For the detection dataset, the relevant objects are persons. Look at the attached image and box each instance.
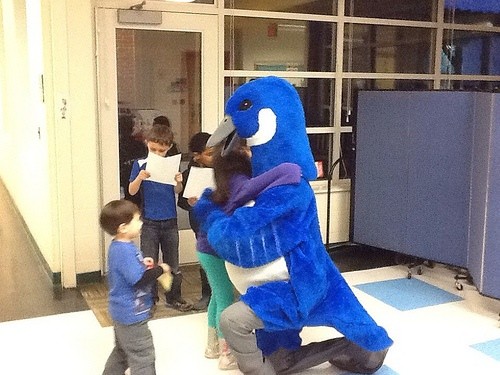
[129,124,194,312]
[119,116,149,209]
[177,132,216,309]
[100,200,171,375]
[153,116,177,156]
[196,151,301,370]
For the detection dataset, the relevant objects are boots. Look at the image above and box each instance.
[219,338,239,371]
[154,280,159,305]
[194,267,211,310]
[204,326,219,359]
[166,271,193,312]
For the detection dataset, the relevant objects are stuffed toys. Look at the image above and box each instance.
[192,76,394,375]
